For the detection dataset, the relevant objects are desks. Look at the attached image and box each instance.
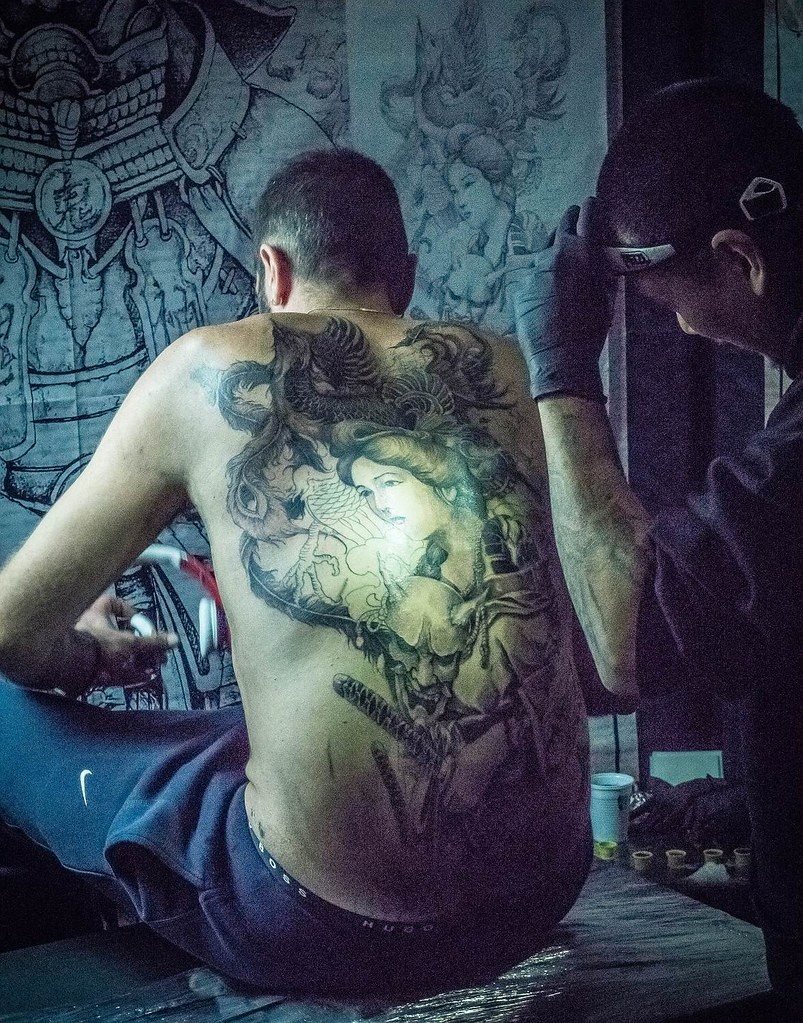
[0,858,784,1023]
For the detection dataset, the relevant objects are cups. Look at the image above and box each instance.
[631,852,653,873]
[734,847,748,872]
[589,773,633,844]
[596,840,617,861]
[703,848,723,865]
[665,849,686,868]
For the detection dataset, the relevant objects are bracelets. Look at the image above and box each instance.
[79,628,103,698]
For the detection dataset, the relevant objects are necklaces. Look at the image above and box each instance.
[307,308,390,315]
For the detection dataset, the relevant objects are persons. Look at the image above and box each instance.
[0,146,594,997]
[512,77,803,1023]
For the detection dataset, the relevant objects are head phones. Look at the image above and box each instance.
[125,544,231,662]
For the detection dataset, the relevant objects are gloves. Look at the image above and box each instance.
[506,196,621,401]
